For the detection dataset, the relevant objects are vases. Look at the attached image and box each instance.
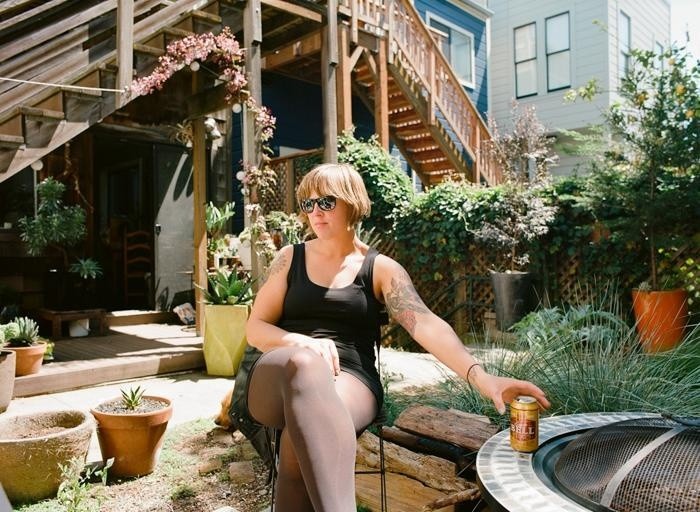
[0,350,92,507]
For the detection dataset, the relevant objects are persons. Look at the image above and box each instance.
[245,162,552,512]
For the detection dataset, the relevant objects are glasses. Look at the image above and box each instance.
[299,195,339,214]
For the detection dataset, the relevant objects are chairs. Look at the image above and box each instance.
[264,302,391,510]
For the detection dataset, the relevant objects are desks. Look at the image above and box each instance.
[477,409,699,511]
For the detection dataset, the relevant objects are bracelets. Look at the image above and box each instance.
[467,361,482,393]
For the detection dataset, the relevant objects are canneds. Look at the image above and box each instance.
[509,396,538,453]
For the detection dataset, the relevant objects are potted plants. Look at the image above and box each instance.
[194,270,259,376]
[0,310,46,373]
[468,92,562,336]
[564,30,699,353]
[91,384,174,477]
